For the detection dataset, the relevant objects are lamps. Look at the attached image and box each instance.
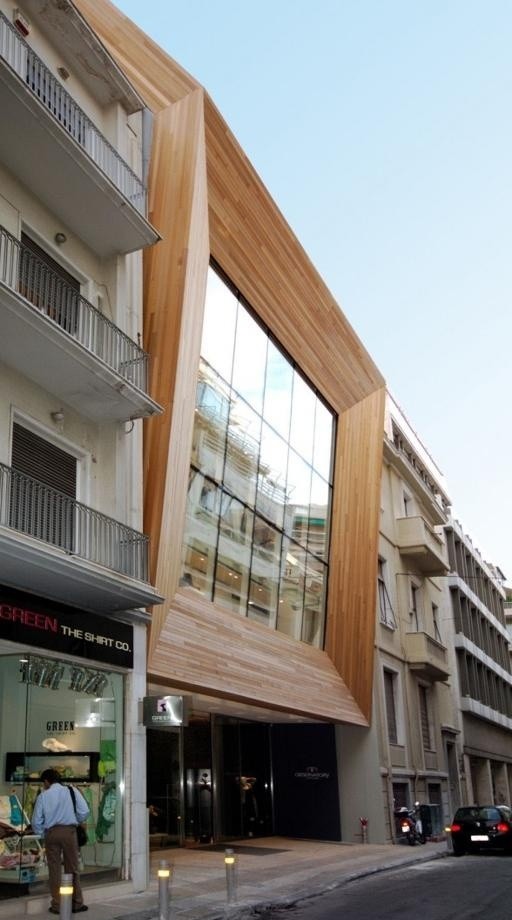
[18,653,109,700]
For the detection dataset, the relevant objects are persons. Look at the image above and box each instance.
[31,769,90,914]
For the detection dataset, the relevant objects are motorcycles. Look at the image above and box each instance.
[394,800,425,847]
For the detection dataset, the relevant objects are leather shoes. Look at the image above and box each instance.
[48,905,88,915]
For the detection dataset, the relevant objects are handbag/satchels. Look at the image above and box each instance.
[76,823,89,847]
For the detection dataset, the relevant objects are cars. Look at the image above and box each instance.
[451,803,512,855]
